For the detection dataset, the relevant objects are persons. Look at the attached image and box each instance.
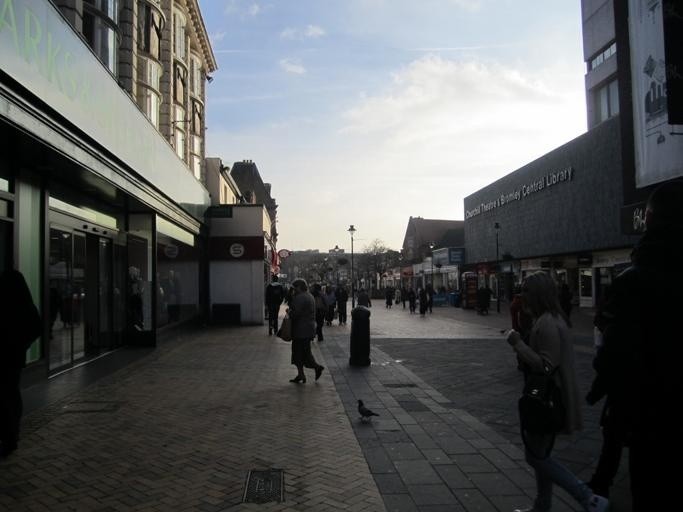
[557,282,572,324]
[383,280,495,316]
[284,279,325,384]
[579,267,631,498]
[507,285,532,373]
[508,270,612,511]
[357,287,371,308]
[284,277,347,344]
[602,178,683,511]
[262,273,282,336]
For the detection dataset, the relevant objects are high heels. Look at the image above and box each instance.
[289,374,307,384]
[315,365,324,381]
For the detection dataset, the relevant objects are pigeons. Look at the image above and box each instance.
[356,400,380,424]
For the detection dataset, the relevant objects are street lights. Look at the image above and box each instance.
[347,225,357,310]
[397,253,402,288]
[313,263,317,281]
[306,265,310,286]
[61,232,68,327]
[334,245,340,287]
[494,222,501,313]
[429,242,434,290]
[323,256,327,285]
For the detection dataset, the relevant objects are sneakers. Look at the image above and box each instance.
[586,495,609,511]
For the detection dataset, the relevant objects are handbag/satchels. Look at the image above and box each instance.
[518,357,565,433]
[276,308,292,342]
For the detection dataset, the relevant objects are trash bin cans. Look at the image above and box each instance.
[349,307,370,366]
[449,293,459,307]
